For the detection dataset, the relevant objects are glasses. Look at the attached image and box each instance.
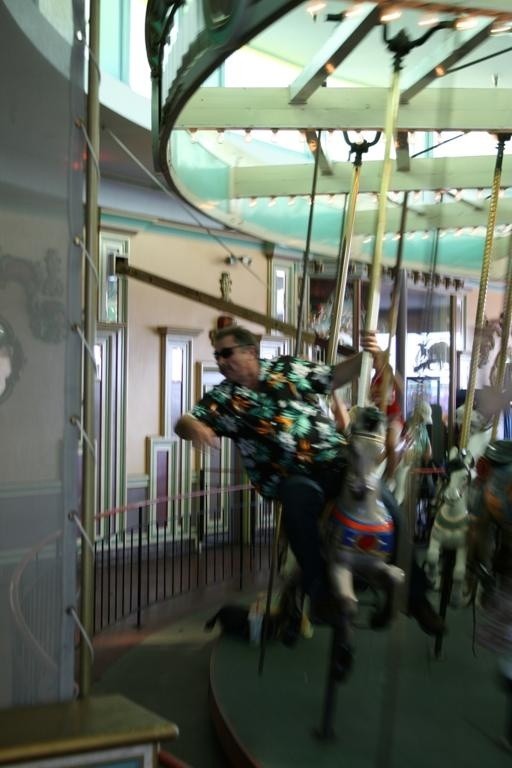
[214,343,253,361]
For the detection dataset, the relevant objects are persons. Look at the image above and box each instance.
[174,325,450,636]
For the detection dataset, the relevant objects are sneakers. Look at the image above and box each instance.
[403,595,448,636]
[330,627,355,683]
